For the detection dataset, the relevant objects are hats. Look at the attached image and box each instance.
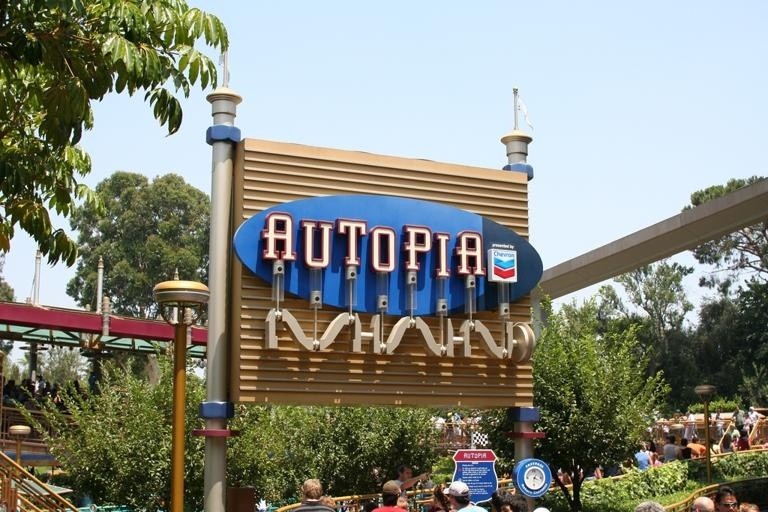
[383,480,400,495]
[444,482,470,496]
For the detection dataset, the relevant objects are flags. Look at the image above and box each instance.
[516,97,536,132]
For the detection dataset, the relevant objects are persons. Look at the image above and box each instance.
[320,479,560,512]
[558,392,768,511]
[430,408,501,446]
[393,463,432,493]
[0,369,102,414]
[289,477,337,512]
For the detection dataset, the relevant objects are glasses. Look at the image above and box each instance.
[720,502,737,507]
